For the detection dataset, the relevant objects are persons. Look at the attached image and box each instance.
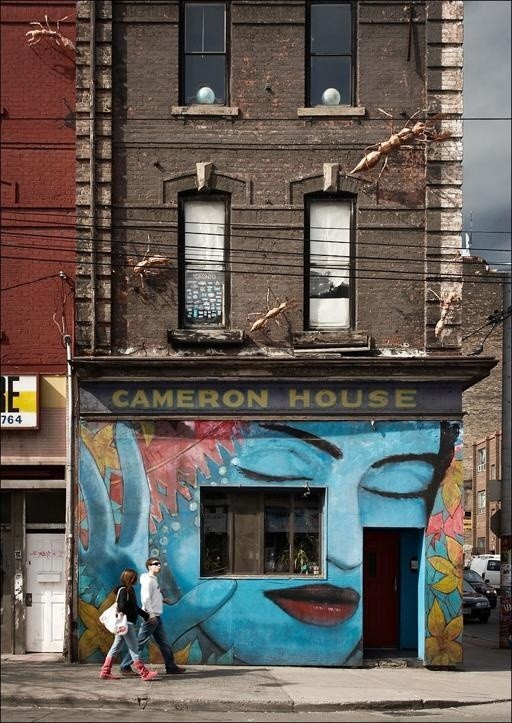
[98,569,158,681]
[119,557,186,676]
[78,420,457,668]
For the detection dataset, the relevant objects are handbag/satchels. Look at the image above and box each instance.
[99,603,128,635]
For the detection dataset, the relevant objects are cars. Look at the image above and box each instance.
[265,545,292,571]
[461,553,502,622]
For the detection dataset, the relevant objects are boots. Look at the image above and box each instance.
[100,657,120,680]
[133,660,158,681]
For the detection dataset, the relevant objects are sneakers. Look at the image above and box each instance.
[121,671,137,676]
[166,668,186,674]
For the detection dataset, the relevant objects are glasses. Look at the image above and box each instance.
[150,563,160,566]
